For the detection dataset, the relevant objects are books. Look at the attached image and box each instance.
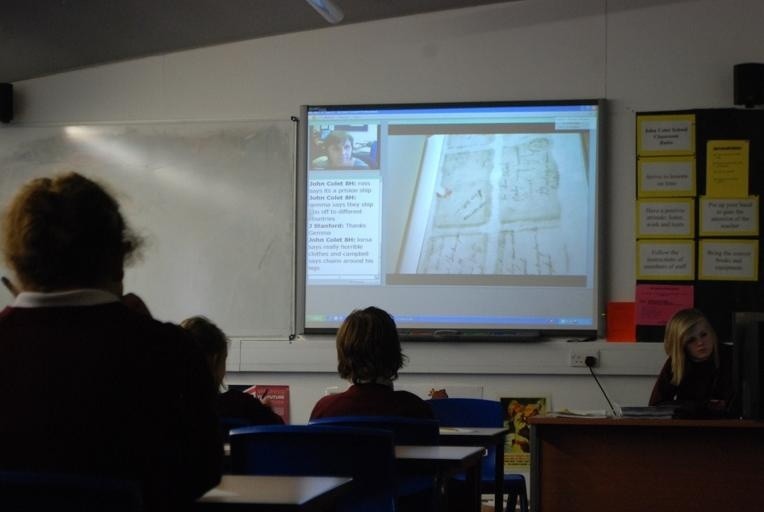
[616,404,672,420]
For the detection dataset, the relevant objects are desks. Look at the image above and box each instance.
[395,445,485,511]
[198,475,354,512]
[438,427,513,512]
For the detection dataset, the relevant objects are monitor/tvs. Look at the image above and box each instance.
[729,308,764,419]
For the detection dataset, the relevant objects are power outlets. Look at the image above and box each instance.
[568,350,601,368]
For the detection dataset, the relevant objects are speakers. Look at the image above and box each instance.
[733,62,764,108]
[0,82,14,124]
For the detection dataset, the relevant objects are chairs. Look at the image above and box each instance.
[306,415,441,512]
[426,399,528,510]
[229,424,395,512]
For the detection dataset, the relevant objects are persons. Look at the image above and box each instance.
[0,168,223,511]
[647,307,736,412]
[178,312,284,439]
[311,306,480,511]
[313,129,370,168]
